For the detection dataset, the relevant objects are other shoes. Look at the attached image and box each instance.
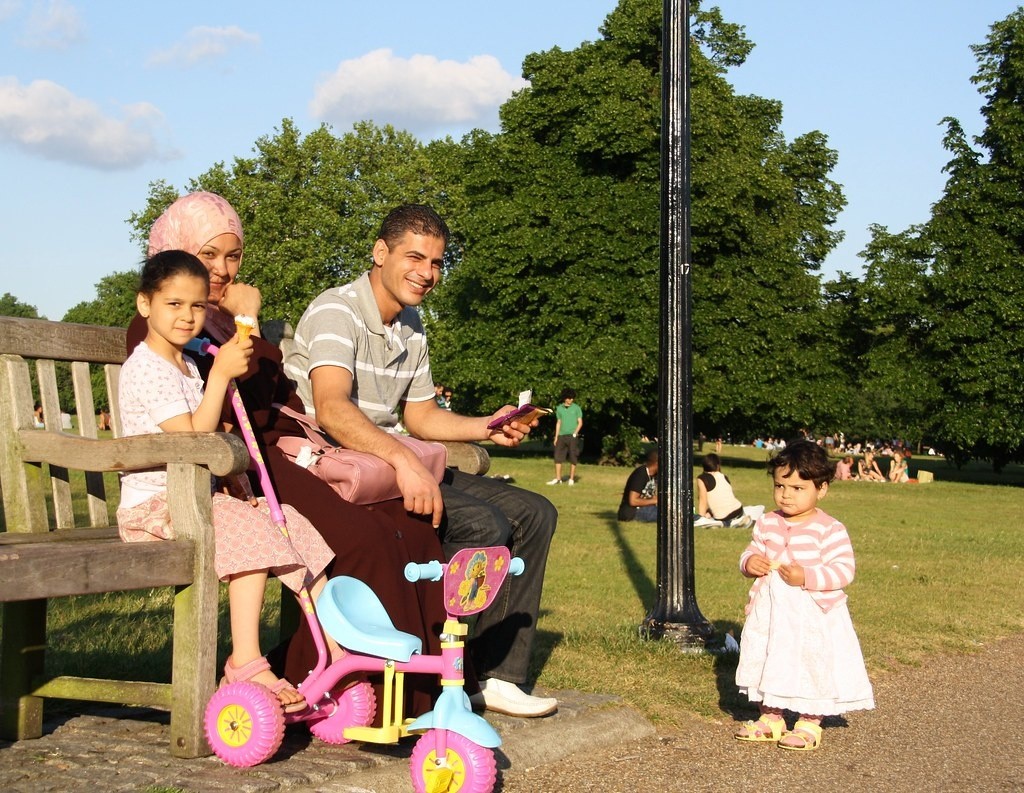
[468,677,557,717]
[546,479,563,485]
[568,479,574,486]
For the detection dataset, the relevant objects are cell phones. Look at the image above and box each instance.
[487,403,550,432]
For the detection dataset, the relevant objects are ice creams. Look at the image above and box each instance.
[235,314,255,342]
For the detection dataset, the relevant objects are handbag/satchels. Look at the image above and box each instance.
[275,432,447,505]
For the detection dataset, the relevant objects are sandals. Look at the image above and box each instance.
[734,715,787,741]
[219,655,307,712]
[777,720,822,751]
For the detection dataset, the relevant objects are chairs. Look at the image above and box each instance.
[0,312,494,767]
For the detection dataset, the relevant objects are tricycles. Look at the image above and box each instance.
[180,336,524,793]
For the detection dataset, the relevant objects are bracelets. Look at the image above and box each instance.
[573,434,577,438]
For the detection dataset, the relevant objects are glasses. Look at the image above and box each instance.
[445,395,451,398]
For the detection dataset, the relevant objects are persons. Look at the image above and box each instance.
[116,250,366,712]
[736,440,876,751]
[125,192,483,728]
[641,432,658,443]
[751,427,945,483]
[715,431,722,453]
[284,202,558,717]
[698,432,706,452]
[546,387,582,486]
[34,403,110,431]
[434,381,453,412]
[617,447,746,530]
